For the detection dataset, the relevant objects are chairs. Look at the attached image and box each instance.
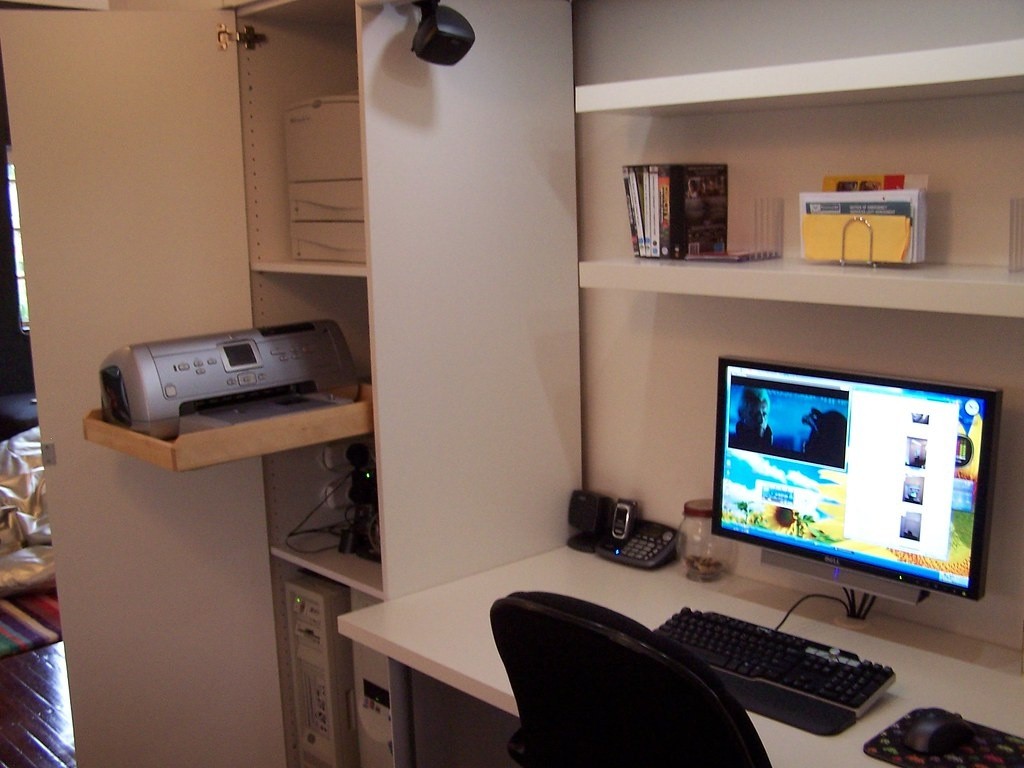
[489,590,772,768]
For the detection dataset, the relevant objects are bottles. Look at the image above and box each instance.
[676,499,731,581]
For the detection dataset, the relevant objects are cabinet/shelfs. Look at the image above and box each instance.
[0,0,585,767]
[574,40,1024,318]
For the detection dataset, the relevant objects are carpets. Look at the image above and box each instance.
[0,586,62,660]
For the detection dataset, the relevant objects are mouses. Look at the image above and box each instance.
[904,709,975,755]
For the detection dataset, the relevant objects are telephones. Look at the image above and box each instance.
[595,498,686,571]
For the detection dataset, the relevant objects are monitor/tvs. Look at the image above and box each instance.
[712,355,1003,603]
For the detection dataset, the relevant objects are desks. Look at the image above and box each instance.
[336,546,1024,768]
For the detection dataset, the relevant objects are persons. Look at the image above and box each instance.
[728,387,772,447]
[801,408,847,463]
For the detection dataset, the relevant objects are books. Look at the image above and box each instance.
[622,164,728,258]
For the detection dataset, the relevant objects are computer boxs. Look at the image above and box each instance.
[286,580,395,768]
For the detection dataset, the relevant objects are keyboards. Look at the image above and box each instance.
[653,607,896,739]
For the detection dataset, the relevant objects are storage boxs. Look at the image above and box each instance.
[286,96,366,265]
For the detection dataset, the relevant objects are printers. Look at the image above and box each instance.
[97,318,360,443]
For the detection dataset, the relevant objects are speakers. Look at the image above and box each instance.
[567,491,614,554]
